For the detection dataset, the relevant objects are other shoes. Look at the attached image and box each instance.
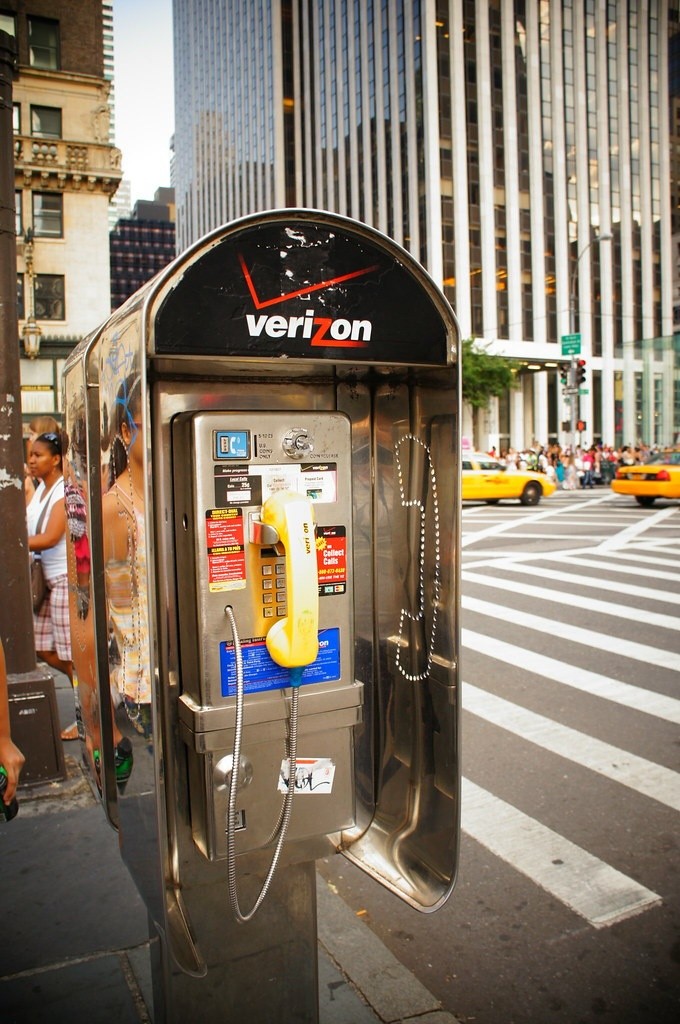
[94,738,132,784]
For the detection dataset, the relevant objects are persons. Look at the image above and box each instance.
[0,638,26,806]
[63,370,151,800]
[490,441,680,489]
[22,416,65,508]
[22,433,78,740]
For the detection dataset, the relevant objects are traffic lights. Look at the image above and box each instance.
[562,421,570,431]
[576,420,586,431]
[560,365,567,384]
[576,360,586,383]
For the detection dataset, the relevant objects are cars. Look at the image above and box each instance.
[462,453,556,506]
[611,448,680,506]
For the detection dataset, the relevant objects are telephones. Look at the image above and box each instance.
[169,406,358,710]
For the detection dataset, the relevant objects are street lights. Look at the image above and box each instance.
[568,233,614,490]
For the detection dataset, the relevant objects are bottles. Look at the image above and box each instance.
[0,766,19,823]
[93,738,133,795]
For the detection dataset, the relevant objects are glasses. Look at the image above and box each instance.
[41,432,61,447]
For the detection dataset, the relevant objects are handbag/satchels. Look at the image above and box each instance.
[30,559,45,615]
